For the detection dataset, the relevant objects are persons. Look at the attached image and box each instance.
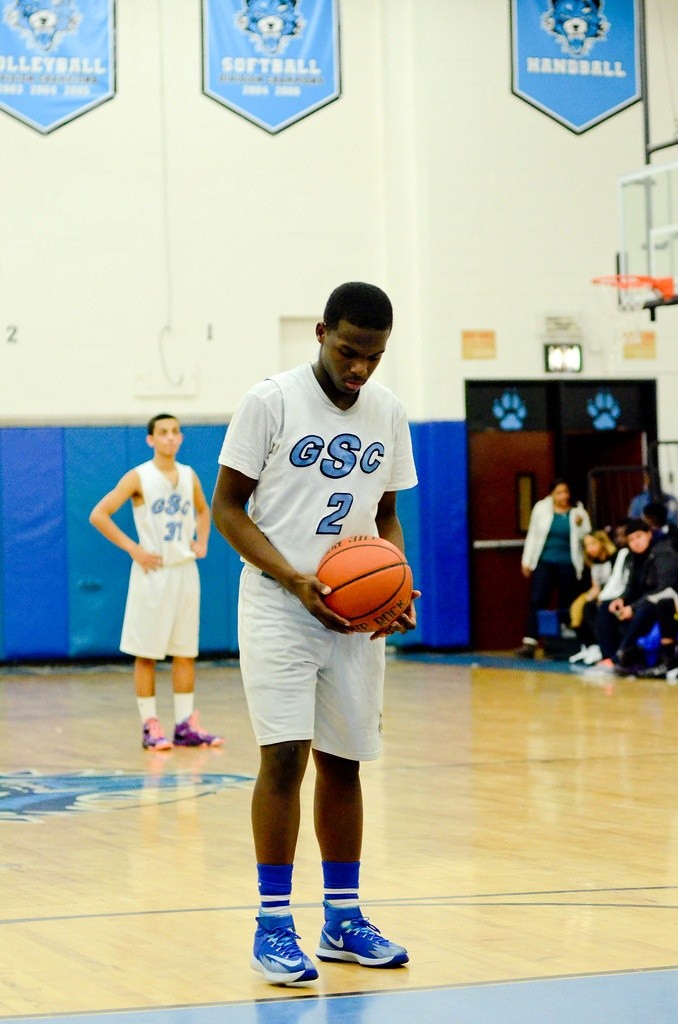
[520,476,678,684]
[88,413,223,753]
[209,281,422,985]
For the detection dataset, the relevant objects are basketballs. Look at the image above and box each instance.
[318,534,414,633]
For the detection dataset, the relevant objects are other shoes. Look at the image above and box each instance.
[522,646,636,681]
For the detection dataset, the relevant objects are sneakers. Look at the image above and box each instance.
[172,710,222,747]
[249,909,318,986]
[315,900,409,967]
[143,718,173,751]
